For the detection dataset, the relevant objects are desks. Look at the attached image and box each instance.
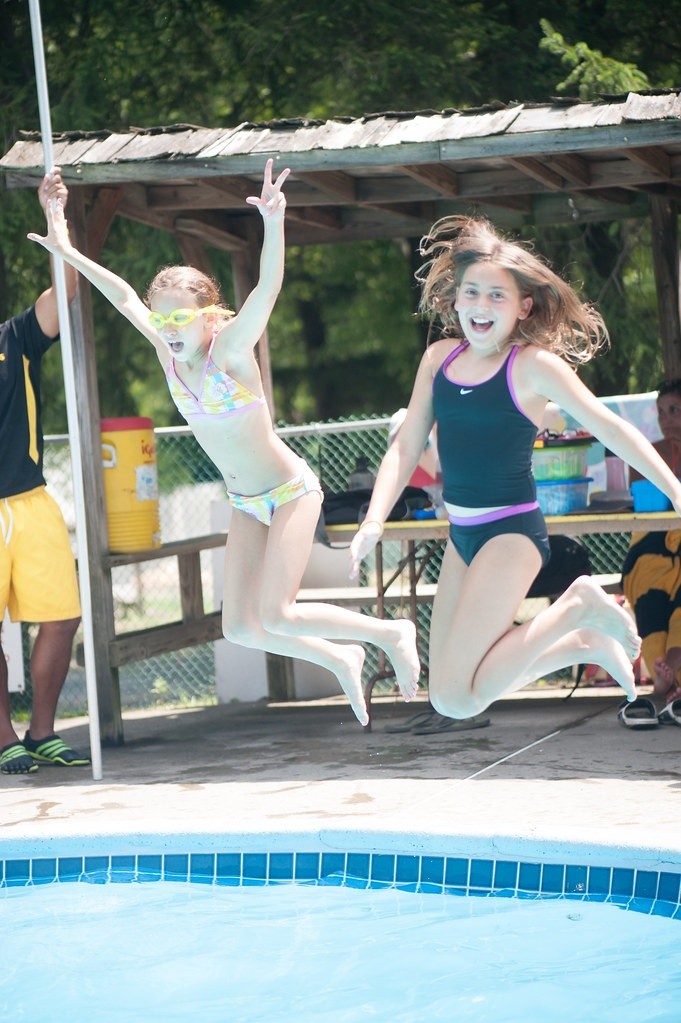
[309,510,681,733]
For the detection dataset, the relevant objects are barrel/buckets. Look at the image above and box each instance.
[100,417,162,553]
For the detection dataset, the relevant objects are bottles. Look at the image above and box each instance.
[604,448,627,493]
[351,459,372,489]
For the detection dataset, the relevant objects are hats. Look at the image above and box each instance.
[386,408,408,447]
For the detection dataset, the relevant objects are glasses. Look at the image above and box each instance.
[149,305,236,330]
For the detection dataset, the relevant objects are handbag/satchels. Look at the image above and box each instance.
[314,484,437,549]
[526,534,592,598]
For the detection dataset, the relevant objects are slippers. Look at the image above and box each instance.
[411,712,490,735]
[384,711,436,734]
[657,698,681,727]
[616,697,660,727]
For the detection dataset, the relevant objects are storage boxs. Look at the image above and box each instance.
[530,443,596,515]
[627,480,671,514]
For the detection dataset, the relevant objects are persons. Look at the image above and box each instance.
[620,379,680,702]
[0,166,92,774]
[28,159,421,727]
[350,216,680,719]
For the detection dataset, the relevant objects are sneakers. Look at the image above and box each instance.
[0,741,39,774]
[21,729,91,767]
